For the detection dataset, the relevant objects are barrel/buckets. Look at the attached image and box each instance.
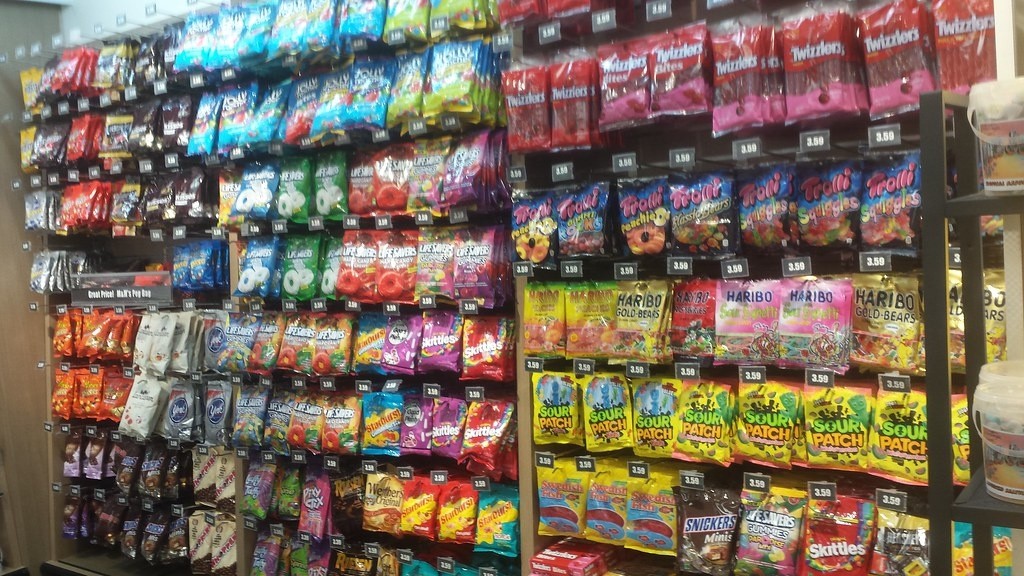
[966,77,1024,195]
[972,360,1024,505]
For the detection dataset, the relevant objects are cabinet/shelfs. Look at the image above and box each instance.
[917,91,1024,576]
